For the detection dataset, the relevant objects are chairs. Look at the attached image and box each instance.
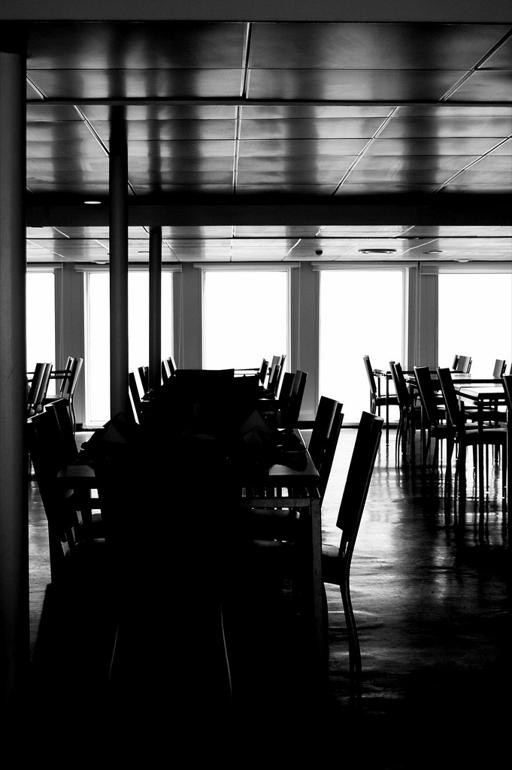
[25,355,381,705]
[361,356,509,537]
